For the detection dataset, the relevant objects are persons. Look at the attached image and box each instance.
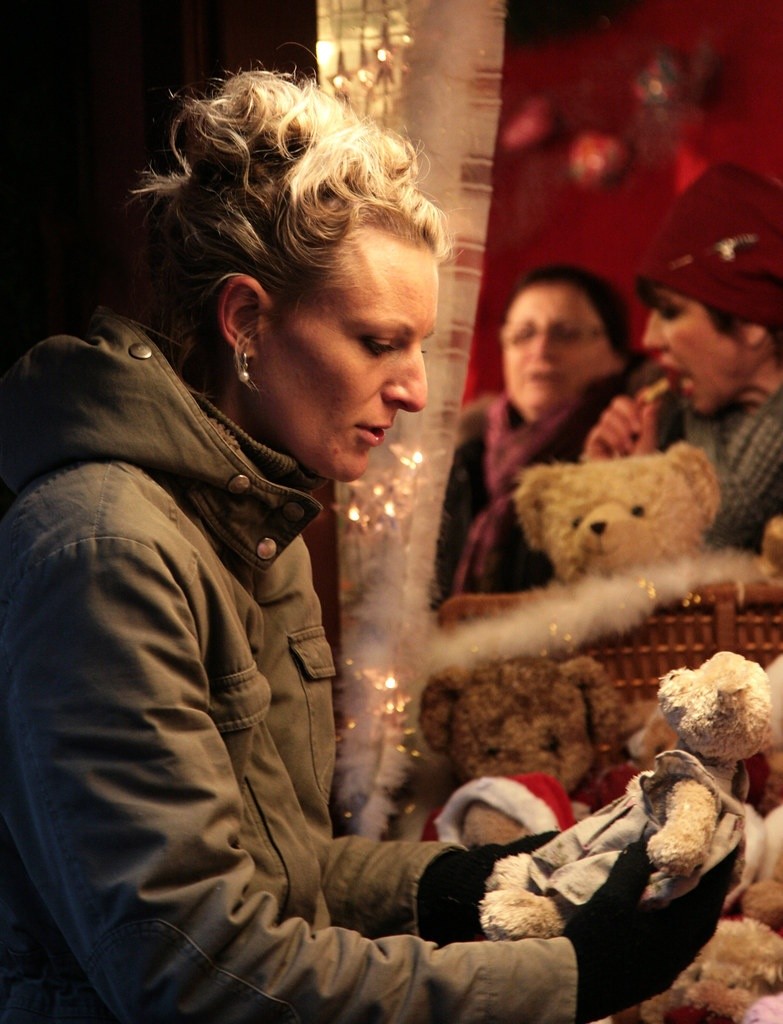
[575,159,783,561]
[421,258,648,614]
[0,70,741,1024]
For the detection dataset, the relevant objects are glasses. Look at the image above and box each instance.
[500,320,605,348]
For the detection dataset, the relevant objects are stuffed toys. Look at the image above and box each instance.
[418,653,783,1024]
[511,440,725,601]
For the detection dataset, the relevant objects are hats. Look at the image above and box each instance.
[633,162,783,329]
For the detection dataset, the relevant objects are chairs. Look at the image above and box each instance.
[445,576,783,704]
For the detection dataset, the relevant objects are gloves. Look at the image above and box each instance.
[557,839,740,1024]
[413,830,567,943]
